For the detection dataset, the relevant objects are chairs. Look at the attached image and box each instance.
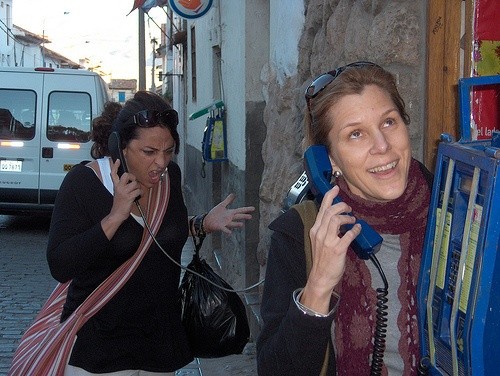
[20,110,34,130]
[56,110,79,128]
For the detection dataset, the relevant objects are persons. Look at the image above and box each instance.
[256,62,445,376]
[47,89,256,376]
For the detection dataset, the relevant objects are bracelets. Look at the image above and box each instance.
[194,213,209,236]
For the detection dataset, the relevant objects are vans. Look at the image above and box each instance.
[0,66,115,216]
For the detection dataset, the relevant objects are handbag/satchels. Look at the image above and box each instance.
[10,280,75,376]
[179,217,249,358]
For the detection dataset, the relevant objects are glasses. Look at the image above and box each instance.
[304,61,382,127]
[120,110,180,131]
